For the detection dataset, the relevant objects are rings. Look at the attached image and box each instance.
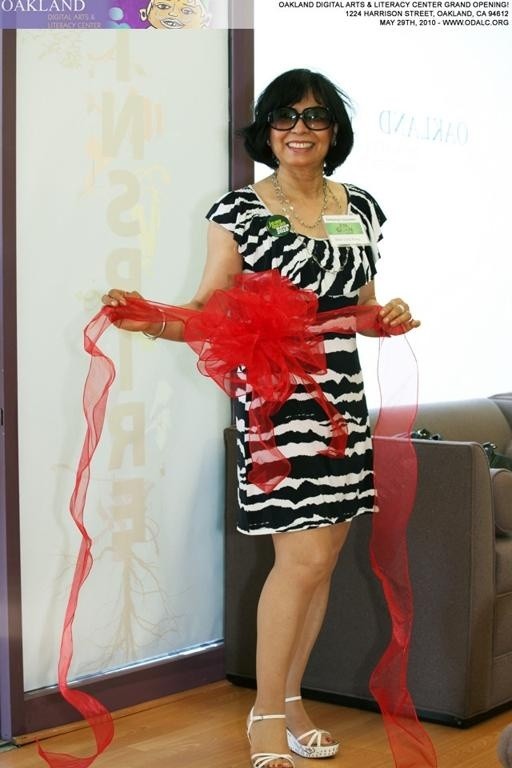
[398,303,405,313]
[406,311,411,320]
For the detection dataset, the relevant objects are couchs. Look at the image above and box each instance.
[222,394,511,730]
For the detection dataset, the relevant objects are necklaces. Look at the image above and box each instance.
[271,172,350,274]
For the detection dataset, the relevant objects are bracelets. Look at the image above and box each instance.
[142,308,168,339]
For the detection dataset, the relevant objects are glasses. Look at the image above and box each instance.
[264,105,335,131]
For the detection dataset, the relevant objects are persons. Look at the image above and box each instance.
[100,69,421,768]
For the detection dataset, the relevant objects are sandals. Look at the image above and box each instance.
[247,706,296,768]
[286,696,340,760]
